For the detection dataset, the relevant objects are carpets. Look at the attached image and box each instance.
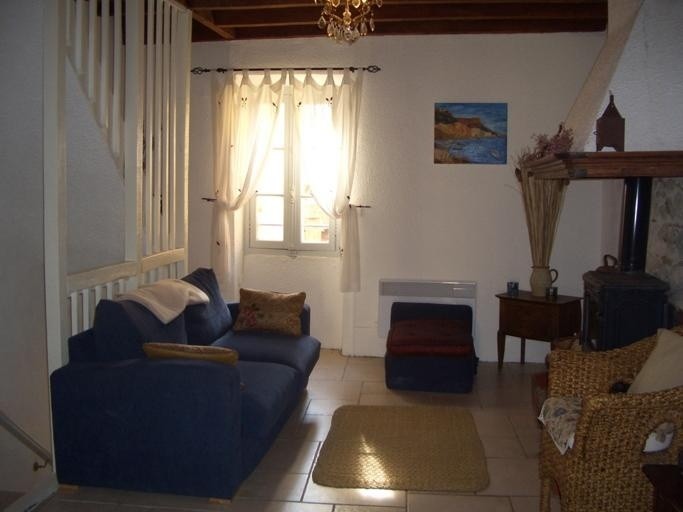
[313,403,490,493]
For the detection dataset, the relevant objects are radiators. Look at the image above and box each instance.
[378,278,478,338]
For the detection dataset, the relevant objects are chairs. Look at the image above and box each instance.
[539,327,683,512]
[384,302,480,393]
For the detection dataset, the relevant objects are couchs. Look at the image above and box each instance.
[50,268,322,500]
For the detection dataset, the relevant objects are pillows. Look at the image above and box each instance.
[141,342,245,392]
[627,328,683,395]
[233,287,309,338]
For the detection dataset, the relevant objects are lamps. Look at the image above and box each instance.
[313,0,387,46]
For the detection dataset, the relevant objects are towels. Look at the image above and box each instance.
[112,278,211,324]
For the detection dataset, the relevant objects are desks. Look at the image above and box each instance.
[642,465,683,512]
[493,288,585,369]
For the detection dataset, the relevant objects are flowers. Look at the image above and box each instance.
[515,129,574,264]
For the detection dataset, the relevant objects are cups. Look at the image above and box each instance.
[506,281,518,297]
[545,286,557,301]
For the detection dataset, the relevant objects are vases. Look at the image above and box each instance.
[530,266,558,298]
